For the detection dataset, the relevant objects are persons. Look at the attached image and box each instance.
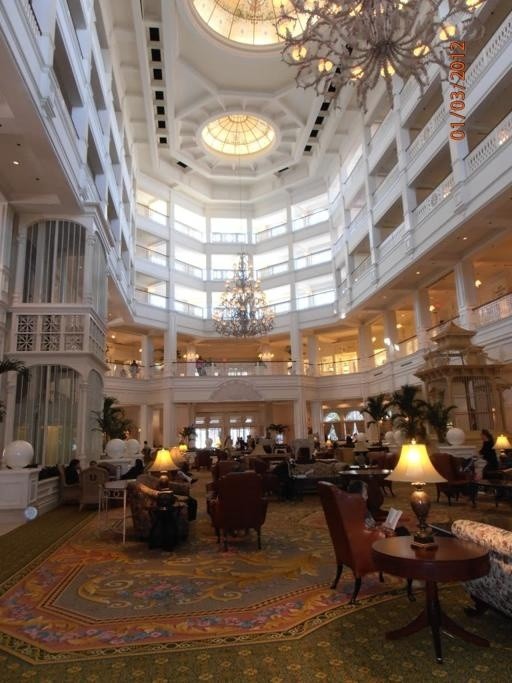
[129,359,139,377]
[120,459,145,479]
[476,428,498,493]
[222,434,256,460]
[90,460,98,466]
[196,355,207,375]
[347,480,411,537]
[143,440,150,448]
[64,458,83,483]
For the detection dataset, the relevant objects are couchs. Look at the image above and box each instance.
[451,520,512,620]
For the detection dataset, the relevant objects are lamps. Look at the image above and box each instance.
[491,434,512,470]
[383,437,449,551]
[210,121,277,339]
[147,448,182,496]
[271,0,487,115]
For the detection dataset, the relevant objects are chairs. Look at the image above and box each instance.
[130,462,190,542]
[429,453,476,506]
[57,461,121,513]
[207,473,267,552]
[206,461,244,528]
[366,452,396,469]
[317,481,416,603]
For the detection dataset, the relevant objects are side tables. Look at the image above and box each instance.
[372,536,490,664]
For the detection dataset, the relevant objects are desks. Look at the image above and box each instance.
[334,469,395,497]
[97,478,137,545]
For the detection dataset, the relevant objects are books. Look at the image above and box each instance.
[381,506,403,530]
